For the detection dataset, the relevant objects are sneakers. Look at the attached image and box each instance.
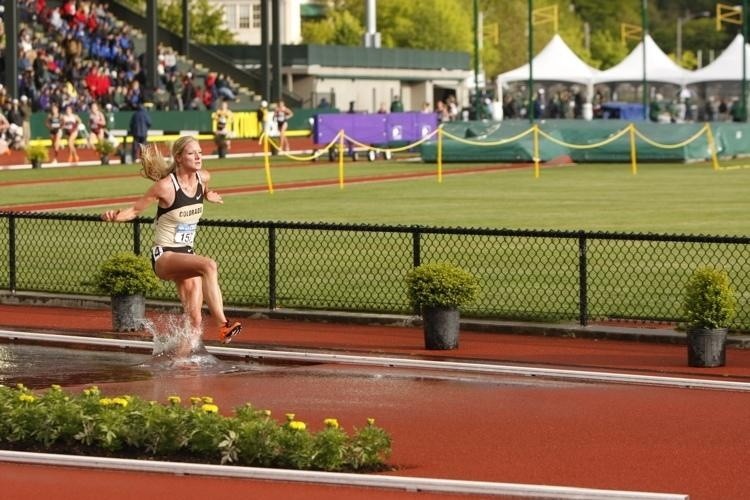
[218,320,244,345]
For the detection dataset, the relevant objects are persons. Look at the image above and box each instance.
[0,0,273,163]
[348,102,357,156]
[390,96,402,113]
[378,104,386,114]
[422,85,604,122]
[100,135,242,359]
[318,99,329,108]
[649,93,749,124]
[271,99,294,152]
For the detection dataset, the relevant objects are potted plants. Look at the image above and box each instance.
[95,138,114,165]
[24,142,48,169]
[95,251,160,331]
[675,267,737,369]
[404,261,480,349]
